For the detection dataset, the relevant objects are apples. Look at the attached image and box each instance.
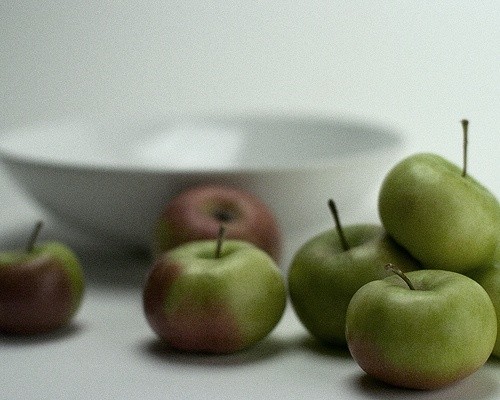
[151,184,281,262]
[286,119,499,390]
[0,219,85,337]
[142,224,287,356]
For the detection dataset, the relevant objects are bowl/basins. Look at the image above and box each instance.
[0,105,404,254]
